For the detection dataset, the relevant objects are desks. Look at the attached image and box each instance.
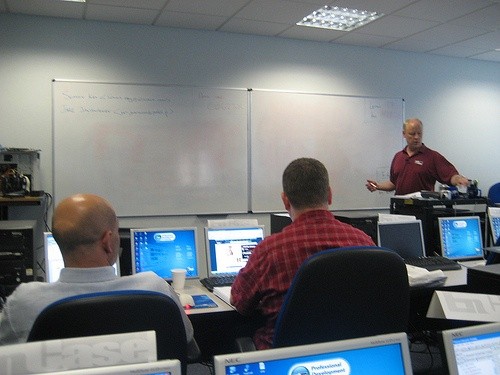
[172,279,248,375]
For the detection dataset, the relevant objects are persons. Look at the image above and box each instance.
[231,157,379,350]
[366,118,469,214]
[0,194,193,347]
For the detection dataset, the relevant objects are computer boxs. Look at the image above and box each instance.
[0,220,37,311]
[390,194,487,257]
[271,213,292,236]
[333,212,378,248]
[467,263,500,297]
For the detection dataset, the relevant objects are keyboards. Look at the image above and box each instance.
[200,276,239,291]
[403,255,459,271]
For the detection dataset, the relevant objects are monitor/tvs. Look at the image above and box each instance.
[441,322,500,375]
[38,359,182,375]
[438,216,483,260]
[489,215,500,245]
[376,219,426,257]
[44,232,120,284]
[130,227,199,281]
[204,225,264,277]
[213,333,414,375]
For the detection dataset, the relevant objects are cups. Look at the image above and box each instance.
[171,269,186,292]
[467,186,481,198]
[445,187,458,200]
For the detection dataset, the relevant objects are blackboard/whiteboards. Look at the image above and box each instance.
[250,88,406,213]
[52,80,250,217]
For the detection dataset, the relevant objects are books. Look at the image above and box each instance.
[213,287,239,312]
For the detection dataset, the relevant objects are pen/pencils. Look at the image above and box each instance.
[370,182,380,190]
